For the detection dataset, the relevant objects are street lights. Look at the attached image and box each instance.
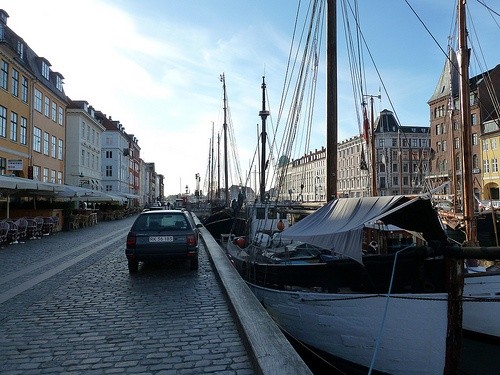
[185,185,189,209]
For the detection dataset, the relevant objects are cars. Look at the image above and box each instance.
[140,199,175,212]
[125,209,203,275]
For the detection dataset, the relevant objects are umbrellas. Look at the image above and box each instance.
[0,174,136,247]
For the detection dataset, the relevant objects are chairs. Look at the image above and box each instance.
[0,205,143,248]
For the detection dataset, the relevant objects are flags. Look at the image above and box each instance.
[362,104,370,144]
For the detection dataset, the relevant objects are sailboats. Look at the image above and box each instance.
[420,1,500,362]
[236,0,469,373]
[199,0,322,244]
